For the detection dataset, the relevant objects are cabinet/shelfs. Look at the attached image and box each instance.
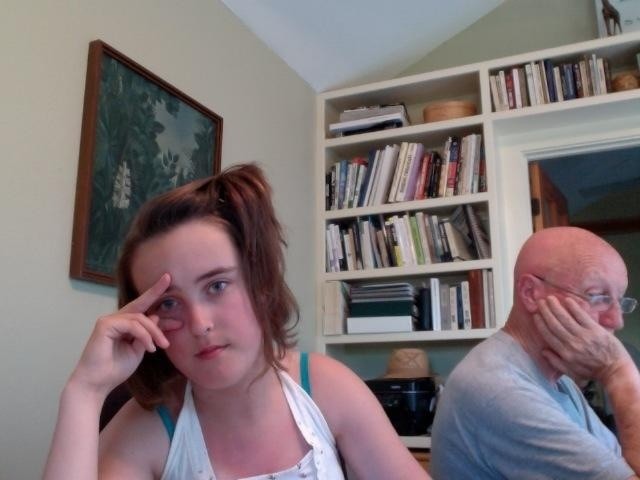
[316,30,640,451]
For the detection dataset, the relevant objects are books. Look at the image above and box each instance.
[490,53,613,111]
[322,101,495,335]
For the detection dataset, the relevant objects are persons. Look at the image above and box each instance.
[430,224,640,480]
[43,158,433,480]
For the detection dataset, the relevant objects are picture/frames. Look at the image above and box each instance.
[70,39,224,285]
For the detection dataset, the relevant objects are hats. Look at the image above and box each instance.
[385,348,438,380]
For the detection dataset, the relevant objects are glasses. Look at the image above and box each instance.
[531,274,637,314]
